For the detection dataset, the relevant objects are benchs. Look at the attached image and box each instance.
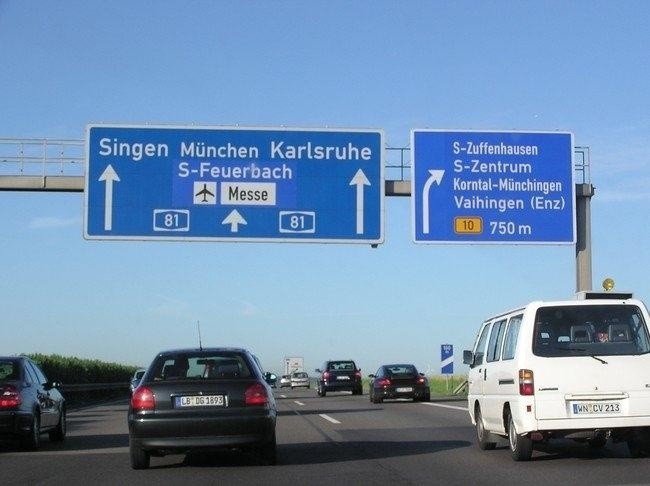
[546,324,636,357]
[163,364,239,380]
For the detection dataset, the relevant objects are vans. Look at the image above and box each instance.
[462,293,650,461]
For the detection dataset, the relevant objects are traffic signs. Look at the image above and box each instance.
[410,128,577,246]
[82,123,385,243]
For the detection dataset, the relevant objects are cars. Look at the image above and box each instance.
[128,348,277,469]
[280,356,430,403]
[1,356,67,450]
